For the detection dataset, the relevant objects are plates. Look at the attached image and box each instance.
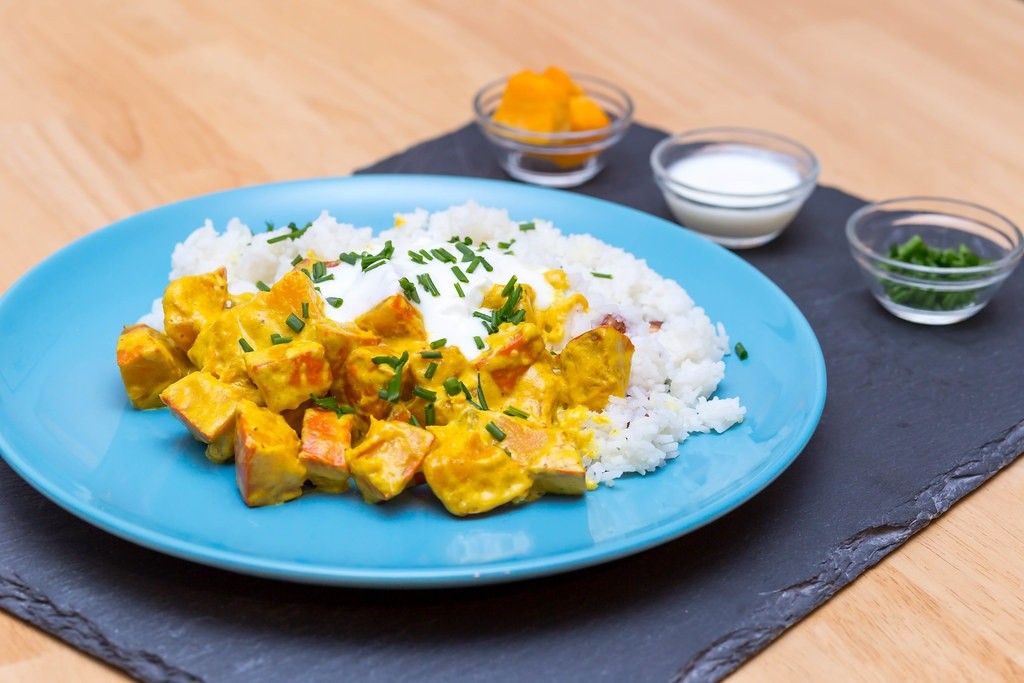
[0,175,829,586]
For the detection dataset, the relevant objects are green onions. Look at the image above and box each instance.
[241,221,749,442]
[876,234,999,310]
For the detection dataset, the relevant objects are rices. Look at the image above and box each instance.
[137,201,746,485]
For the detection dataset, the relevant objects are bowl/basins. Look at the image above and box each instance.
[473,68,635,188]
[844,196,1024,325]
[650,126,820,249]
